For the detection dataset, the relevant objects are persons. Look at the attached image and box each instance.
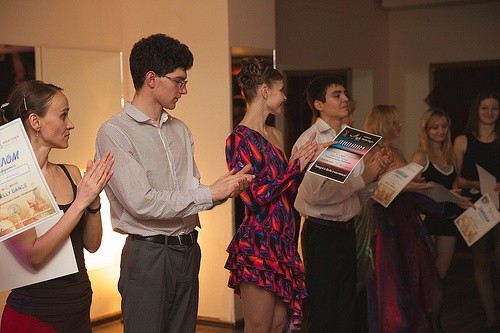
[452,90,500,333]
[225,54,338,333]
[409,107,480,289]
[361,105,445,333]
[93,33,257,333]
[290,75,393,333]
[0,79,116,332]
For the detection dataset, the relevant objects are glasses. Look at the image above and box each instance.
[145,70,187,88]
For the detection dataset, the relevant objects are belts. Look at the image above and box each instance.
[305,215,354,232]
[127,230,198,245]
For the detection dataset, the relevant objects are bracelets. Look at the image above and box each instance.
[86,204,101,213]
